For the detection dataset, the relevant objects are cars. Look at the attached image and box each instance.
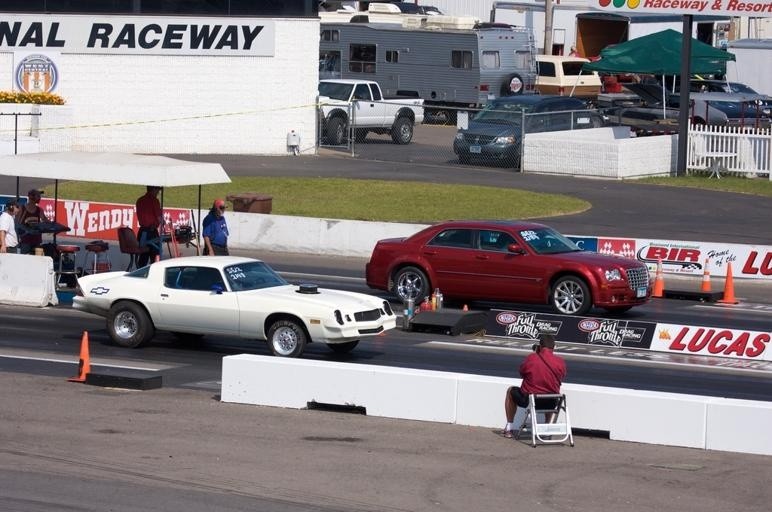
[361,219,656,325]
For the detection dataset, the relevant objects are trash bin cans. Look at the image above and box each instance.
[226,193,273,214]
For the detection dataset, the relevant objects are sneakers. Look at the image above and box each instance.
[498,429,514,438]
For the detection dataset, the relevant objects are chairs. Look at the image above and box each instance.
[117,227,149,272]
[516,394,575,448]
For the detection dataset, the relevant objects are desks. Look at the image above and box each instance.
[695,152,738,179]
[15,222,70,244]
[159,232,199,258]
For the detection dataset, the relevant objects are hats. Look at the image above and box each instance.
[147,185,164,191]
[27,189,45,197]
[5,197,21,208]
[209,198,225,209]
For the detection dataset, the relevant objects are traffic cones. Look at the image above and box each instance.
[68,329,97,381]
[650,257,666,297]
[713,260,745,306]
[701,258,712,294]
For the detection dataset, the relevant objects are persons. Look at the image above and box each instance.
[568,46,582,57]
[0,202,21,254]
[202,199,230,256]
[500,334,567,438]
[14,189,50,255]
[136,186,163,268]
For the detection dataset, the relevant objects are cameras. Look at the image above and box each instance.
[532,344,540,351]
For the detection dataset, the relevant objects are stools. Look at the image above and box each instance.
[81,240,111,277]
[55,245,81,292]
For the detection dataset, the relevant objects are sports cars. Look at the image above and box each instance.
[72,255,402,357]
